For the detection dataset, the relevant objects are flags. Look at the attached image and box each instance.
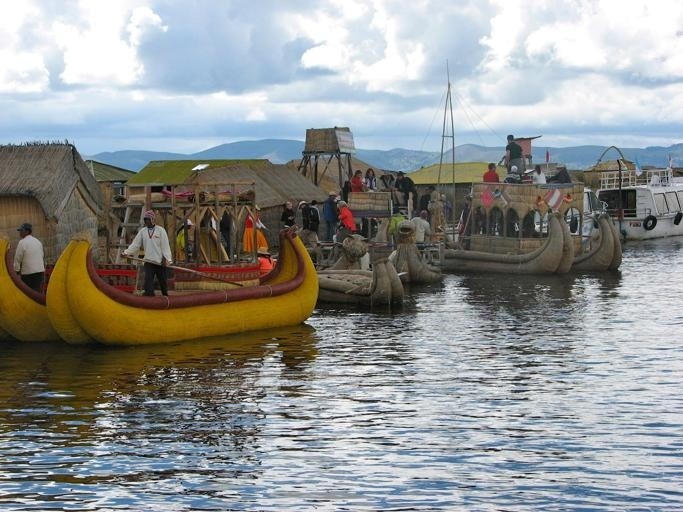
[635,158,643,177]
[667,154,672,171]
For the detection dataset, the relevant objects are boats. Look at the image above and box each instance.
[43,228,319,348]
[305,258,405,309]
[596,187,682,242]
[0,235,61,344]
[468,214,623,272]
[312,233,367,270]
[391,222,443,281]
[440,214,573,275]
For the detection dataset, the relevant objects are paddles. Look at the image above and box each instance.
[345,272,409,293]
[125,255,245,288]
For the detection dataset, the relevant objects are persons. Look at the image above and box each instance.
[456,134,571,240]
[120,209,172,296]
[279,166,453,259]
[175,204,269,264]
[13,223,44,292]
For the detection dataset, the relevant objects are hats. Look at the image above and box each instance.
[382,170,391,175]
[395,171,406,174]
[257,247,270,254]
[556,163,564,168]
[17,223,32,231]
[298,201,307,209]
[329,192,337,196]
[144,211,155,225]
[183,219,193,225]
[511,166,517,172]
